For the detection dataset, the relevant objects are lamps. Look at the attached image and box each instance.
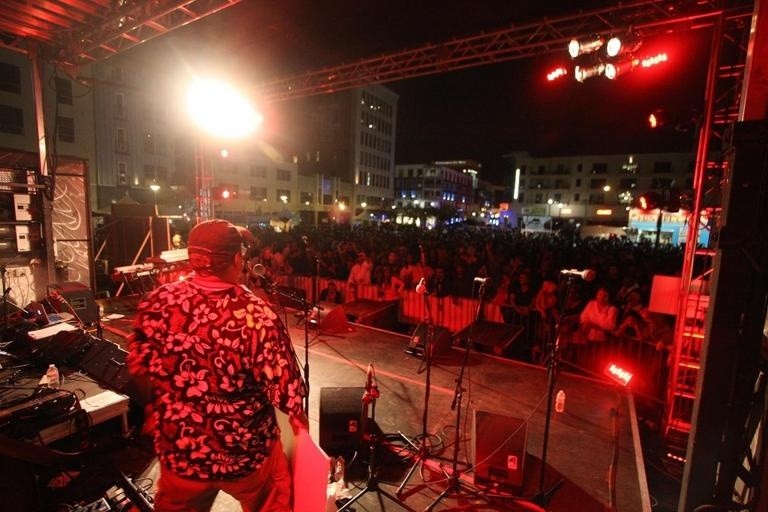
[567,30,644,83]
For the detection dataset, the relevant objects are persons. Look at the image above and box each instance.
[258,219,707,359]
[127,217,310,512]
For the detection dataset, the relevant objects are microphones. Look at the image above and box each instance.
[253,264,272,284]
[415,277,426,294]
[473,276,492,286]
[561,268,596,281]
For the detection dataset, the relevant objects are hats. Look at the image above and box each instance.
[187,219,254,258]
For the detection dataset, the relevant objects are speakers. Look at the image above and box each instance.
[56,281,99,326]
[319,386,371,456]
[472,409,529,496]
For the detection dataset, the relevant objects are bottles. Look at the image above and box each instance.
[46,364,60,389]
[555,389,567,413]
[26,169,36,195]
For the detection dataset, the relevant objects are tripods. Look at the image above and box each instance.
[533,357,564,507]
[423,384,511,512]
[304,262,346,347]
[334,397,419,512]
[396,318,472,496]
